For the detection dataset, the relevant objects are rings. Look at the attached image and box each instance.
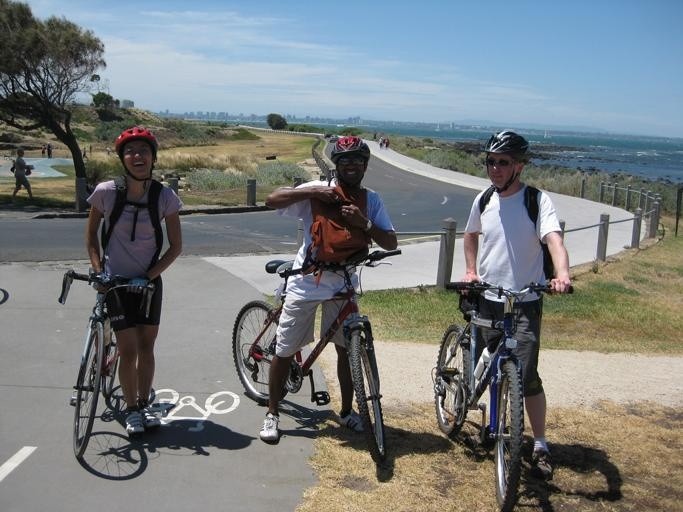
[334,196,338,200]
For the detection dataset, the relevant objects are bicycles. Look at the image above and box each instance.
[232,249,399,464]
[431,280,573,511]
[57,259,156,459]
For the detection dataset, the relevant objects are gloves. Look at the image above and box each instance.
[127,275,150,294]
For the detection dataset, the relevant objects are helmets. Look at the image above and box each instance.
[116,127,159,154]
[331,136,370,162]
[485,131,531,162]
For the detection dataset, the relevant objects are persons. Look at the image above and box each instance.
[82,148,88,161]
[12,149,34,198]
[260,136,397,444]
[462,131,571,479]
[47,142,53,158]
[84,128,184,436]
[374,134,390,150]
[41,144,46,158]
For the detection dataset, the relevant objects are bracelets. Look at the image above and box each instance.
[465,270,478,275]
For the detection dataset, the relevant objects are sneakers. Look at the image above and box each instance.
[141,407,161,428]
[260,412,279,442]
[125,411,145,434]
[339,408,365,431]
[532,449,553,480]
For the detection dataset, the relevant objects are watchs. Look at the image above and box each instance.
[363,219,372,232]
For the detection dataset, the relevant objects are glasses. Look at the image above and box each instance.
[486,157,511,166]
[338,155,364,166]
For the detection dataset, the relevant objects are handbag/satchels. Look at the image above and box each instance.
[312,204,368,262]
